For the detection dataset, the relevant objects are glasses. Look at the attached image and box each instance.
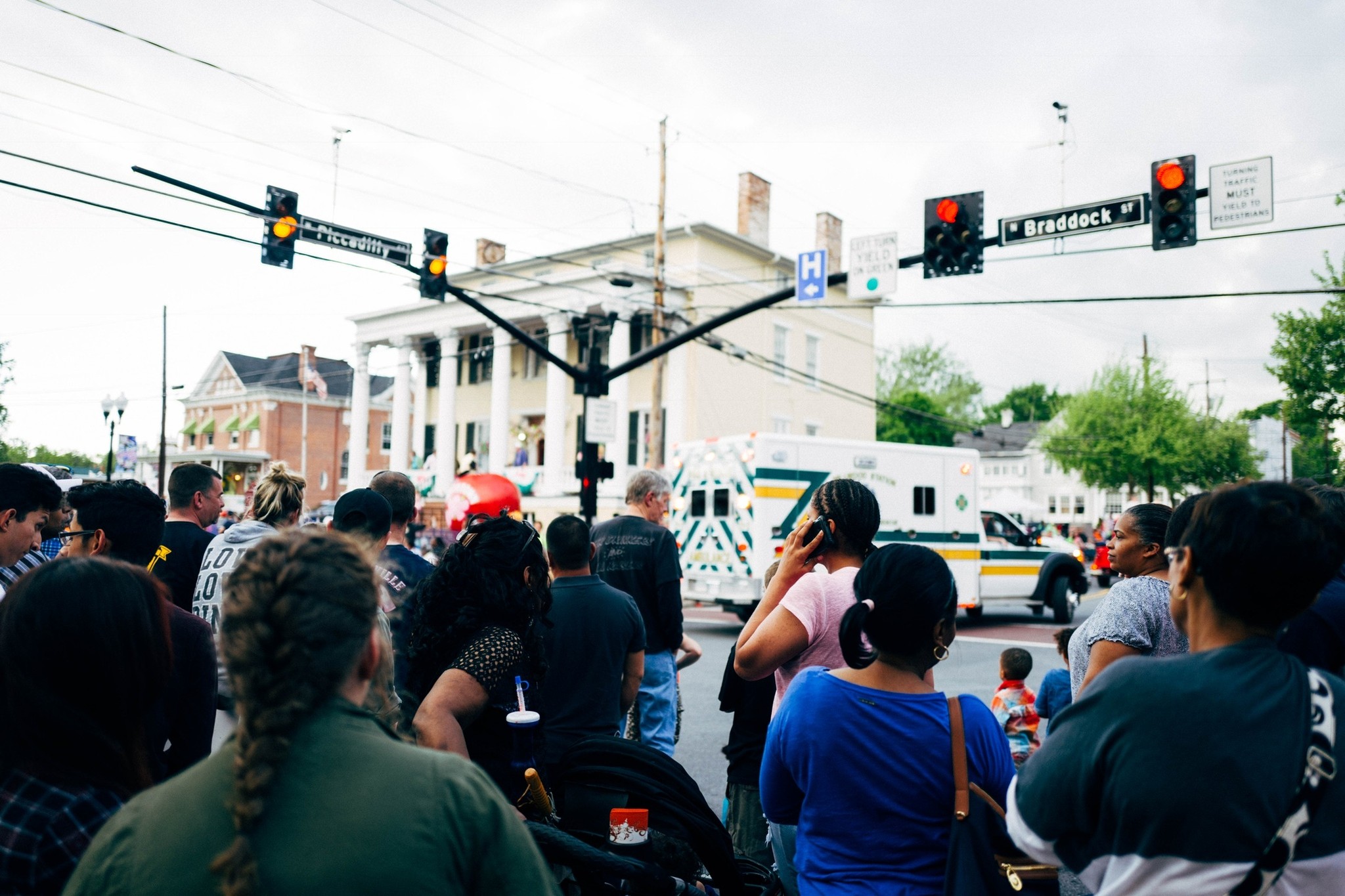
[522,519,542,553]
[1162,544,1183,564]
[58,529,95,547]
[37,463,71,472]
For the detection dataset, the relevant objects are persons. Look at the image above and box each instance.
[1004,480,1345,896]
[0,557,174,896]
[716,479,881,896]
[992,478,1345,770]
[62,527,563,896]
[0,441,702,822]
[760,543,1026,896]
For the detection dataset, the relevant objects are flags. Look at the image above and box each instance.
[298,345,327,400]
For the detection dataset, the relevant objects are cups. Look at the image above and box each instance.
[506,711,540,728]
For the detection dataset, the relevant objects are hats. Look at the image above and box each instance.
[332,487,392,539]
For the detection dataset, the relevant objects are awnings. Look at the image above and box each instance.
[193,419,214,433]
[218,416,240,431]
[239,414,259,430]
[178,421,196,434]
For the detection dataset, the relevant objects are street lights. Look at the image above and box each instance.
[100,390,128,482]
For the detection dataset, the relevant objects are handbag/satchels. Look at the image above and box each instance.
[946,697,1062,896]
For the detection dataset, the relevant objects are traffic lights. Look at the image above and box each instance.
[1150,154,1197,251]
[421,228,449,303]
[261,185,298,270]
[922,190,984,279]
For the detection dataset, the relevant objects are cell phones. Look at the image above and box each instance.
[803,515,835,561]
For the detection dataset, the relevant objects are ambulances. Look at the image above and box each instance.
[660,428,1095,636]
